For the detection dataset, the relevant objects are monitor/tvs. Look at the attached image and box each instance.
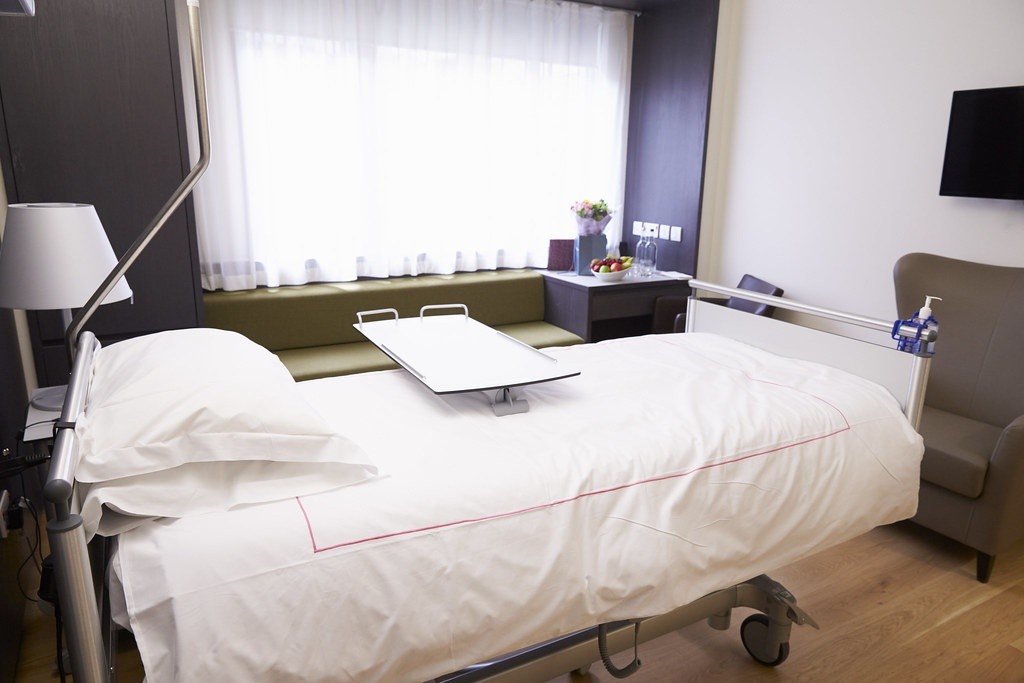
[938,84,1024,200]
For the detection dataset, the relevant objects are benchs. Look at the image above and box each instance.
[204,272,586,385]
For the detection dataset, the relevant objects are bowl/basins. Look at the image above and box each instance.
[591,266,630,281]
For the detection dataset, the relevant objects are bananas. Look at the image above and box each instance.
[620,256,634,268]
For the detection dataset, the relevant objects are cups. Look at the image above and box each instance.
[628,264,641,277]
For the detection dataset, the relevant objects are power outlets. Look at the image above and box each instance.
[0,490,11,538]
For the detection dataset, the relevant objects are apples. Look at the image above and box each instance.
[599,263,622,272]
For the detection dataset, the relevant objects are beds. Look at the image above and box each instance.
[42,281,944,683]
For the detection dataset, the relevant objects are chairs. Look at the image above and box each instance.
[894,252,1024,582]
[674,274,785,333]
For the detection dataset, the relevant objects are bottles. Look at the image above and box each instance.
[644,229,658,276]
[636,228,648,276]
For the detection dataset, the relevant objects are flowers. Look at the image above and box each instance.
[570,199,613,237]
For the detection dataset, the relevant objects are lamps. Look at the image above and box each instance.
[0,203,134,409]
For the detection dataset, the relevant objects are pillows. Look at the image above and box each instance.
[67,326,377,536]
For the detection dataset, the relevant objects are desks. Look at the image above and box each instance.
[541,267,692,339]
